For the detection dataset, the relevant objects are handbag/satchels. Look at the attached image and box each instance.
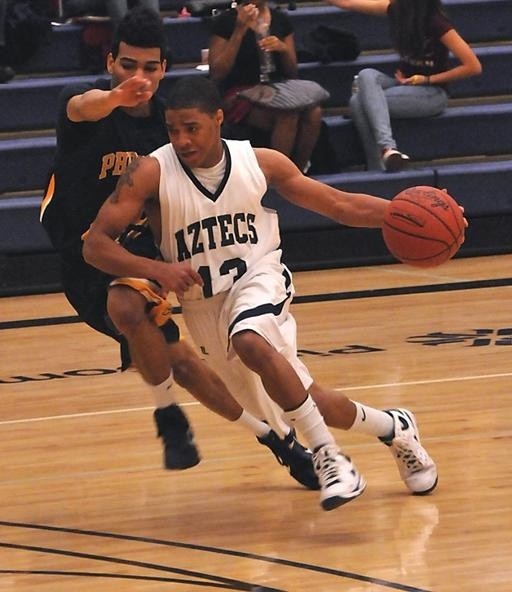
[303,26,362,61]
[236,79,331,114]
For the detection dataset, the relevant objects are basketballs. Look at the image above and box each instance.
[384,187,463,268]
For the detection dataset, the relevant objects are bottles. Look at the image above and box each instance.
[351,75,359,96]
[254,17,277,74]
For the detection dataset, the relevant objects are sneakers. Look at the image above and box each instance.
[380,147,411,170]
[311,443,367,511]
[377,408,438,495]
[256,420,325,490]
[154,401,200,469]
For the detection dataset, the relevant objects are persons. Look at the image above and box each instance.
[82,73,468,513]
[39,8,321,494]
[1,0,160,82]
[330,0,481,172]
[207,0,330,166]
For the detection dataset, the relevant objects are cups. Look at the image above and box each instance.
[201,48,210,66]
